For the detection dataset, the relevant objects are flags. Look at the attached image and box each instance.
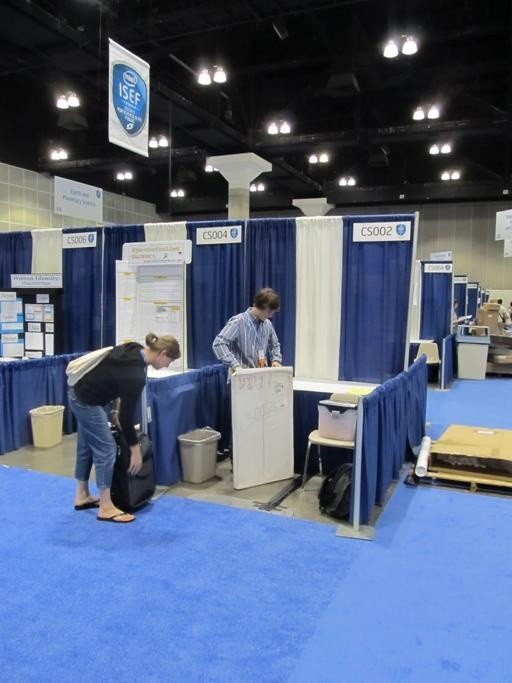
[108,36,153,155]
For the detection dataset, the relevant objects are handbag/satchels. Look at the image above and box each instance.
[318,463,353,520]
[65,346,114,386]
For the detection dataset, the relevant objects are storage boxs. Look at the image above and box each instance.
[477,304,500,335]
[317,400,358,441]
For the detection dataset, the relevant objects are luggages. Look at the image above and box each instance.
[109,424,156,510]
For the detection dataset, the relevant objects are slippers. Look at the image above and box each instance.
[75,499,100,510]
[97,512,136,522]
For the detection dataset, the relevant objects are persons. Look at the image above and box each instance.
[452,298,467,334]
[210,285,284,478]
[496,299,507,322]
[507,300,512,321]
[66,332,181,523]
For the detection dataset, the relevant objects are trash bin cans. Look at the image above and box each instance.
[455,325,491,380]
[29,405,65,449]
[177,428,222,484]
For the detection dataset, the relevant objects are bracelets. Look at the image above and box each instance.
[110,408,120,414]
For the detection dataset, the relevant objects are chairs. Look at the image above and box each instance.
[302,393,361,490]
[414,344,442,389]
